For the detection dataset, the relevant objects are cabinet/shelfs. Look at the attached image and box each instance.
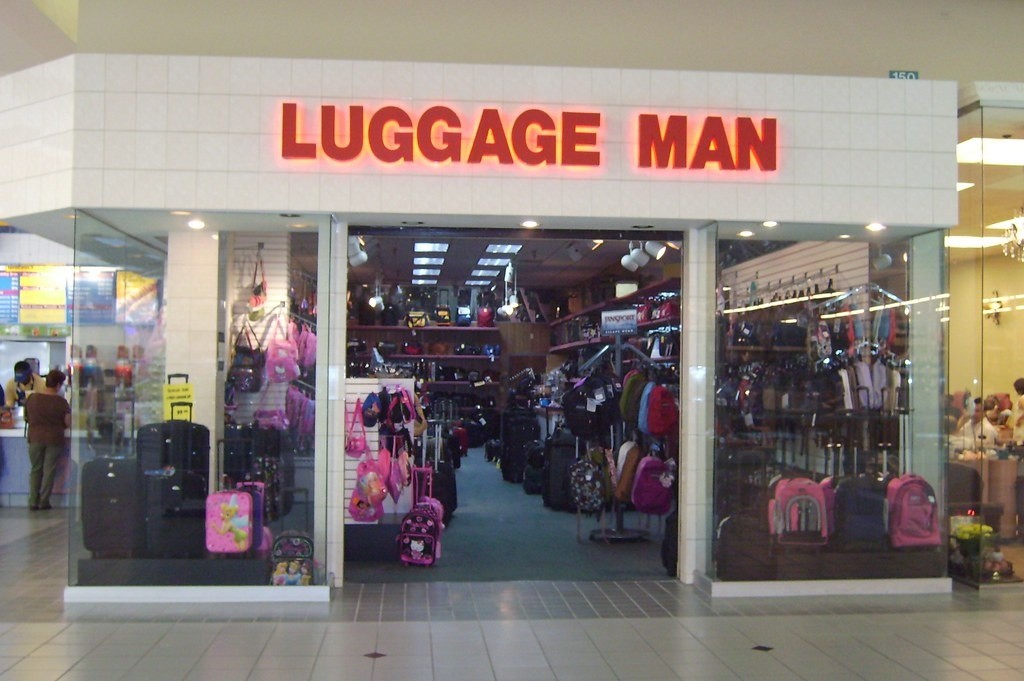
[223,277,898,538]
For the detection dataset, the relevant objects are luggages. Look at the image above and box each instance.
[500,414,540,484]
[887,408,941,549]
[395,466,437,569]
[542,405,576,511]
[270,488,313,585]
[138,401,210,556]
[137,420,209,485]
[350,285,495,328]
[768,408,829,547]
[522,439,542,494]
[719,450,766,581]
[418,396,468,517]
[834,408,887,546]
[81,395,137,559]
[207,438,257,560]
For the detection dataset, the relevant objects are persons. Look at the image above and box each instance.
[1013,379,1024,446]
[959,398,999,445]
[5,361,47,409]
[24,370,72,511]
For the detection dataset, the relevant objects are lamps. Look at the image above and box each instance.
[872,245,892,270]
[621,241,683,272]
[347,234,369,269]
[498,263,519,317]
[369,281,385,311]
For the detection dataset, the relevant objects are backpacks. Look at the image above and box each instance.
[620,349,675,439]
[612,428,671,516]
[562,356,616,522]
[717,341,843,422]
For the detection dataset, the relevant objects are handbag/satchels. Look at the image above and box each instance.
[485,438,501,463]
[632,291,680,324]
[350,431,411,523]
[341,391,363,456]
[463,406,500,449]
[225,240,319,522]
[375,340,503,361]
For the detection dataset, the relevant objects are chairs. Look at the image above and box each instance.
[946,390,1020,540]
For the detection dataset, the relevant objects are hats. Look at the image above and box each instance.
[15,368,33,383]
[364,392,381,427]
